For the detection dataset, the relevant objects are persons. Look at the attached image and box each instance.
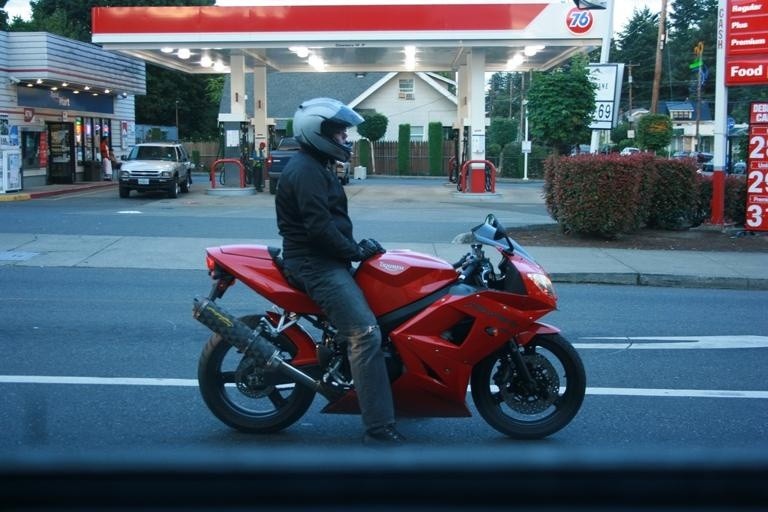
[275,98,408,445]
[696,151,705,170]
[570,145,585,157]
[100,136,117,181]
[448,155,456,181]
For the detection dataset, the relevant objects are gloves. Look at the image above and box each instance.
[356,238,386,262]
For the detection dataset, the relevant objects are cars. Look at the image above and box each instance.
[117,141,194,200]
[567,142,747,175]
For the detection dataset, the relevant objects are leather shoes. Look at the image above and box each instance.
[360,425,409,449]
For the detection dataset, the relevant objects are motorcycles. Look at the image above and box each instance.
[191,211,589,439]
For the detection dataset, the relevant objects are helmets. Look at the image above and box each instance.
[293,96,366,163]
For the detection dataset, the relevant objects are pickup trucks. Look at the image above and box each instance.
[266,135,354,195]
